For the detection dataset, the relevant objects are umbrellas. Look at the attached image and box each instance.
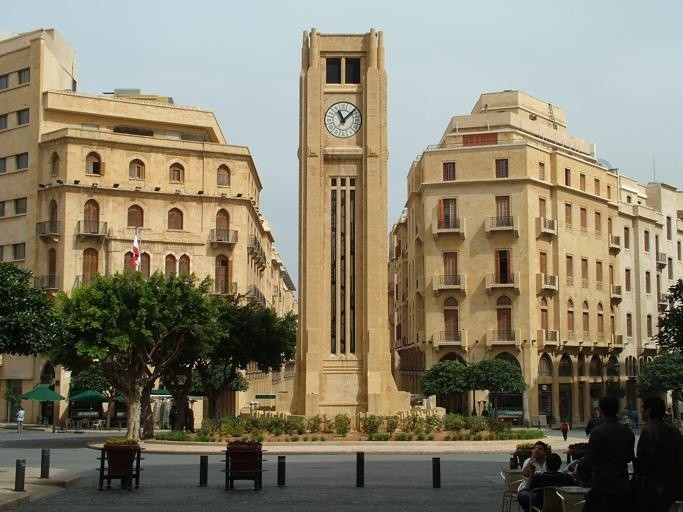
[111,391,155,403]
[15,387,64,428]
[69,389,109,430]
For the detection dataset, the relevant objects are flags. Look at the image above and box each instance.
[130,233,141,268]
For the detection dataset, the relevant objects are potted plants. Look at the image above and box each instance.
[102,430,141,475]
[512,439,554,471]
[568,440,591,466]
[224,431,264,478]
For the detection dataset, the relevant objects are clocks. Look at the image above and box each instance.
[323,102,362,139]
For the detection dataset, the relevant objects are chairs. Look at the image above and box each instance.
[498,470,541,512]
[555,487,587,512]
[62,414,115,431]
[529,480,555,512]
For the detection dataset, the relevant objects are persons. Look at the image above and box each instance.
[628,395,682,512]
[579,396,634,512]
[15,408,25,434]
[584,406,682,439]
[168,404,195,434]
[561,453,585,487]
[516,441,547,512]
[528,454,574,512]
[480,407,488,417]
[560,420,568,442]
